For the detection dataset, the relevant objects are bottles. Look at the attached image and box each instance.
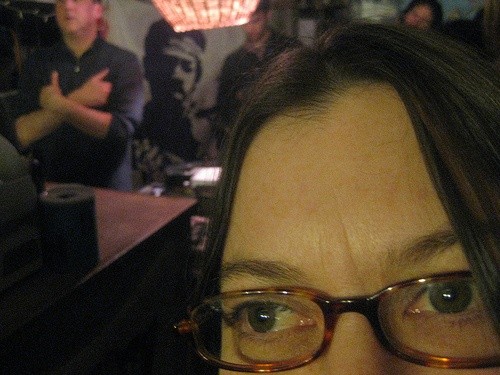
[159,161,184,184]
[164,164,177,195]
[183,180,192,197]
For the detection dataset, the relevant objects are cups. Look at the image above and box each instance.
[154,188,163,197]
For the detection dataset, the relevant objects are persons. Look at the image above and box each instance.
[5,0,144,193]
[216,0,500,155]
[171,20,500,375]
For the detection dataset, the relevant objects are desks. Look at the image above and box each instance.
[0,182,201,375]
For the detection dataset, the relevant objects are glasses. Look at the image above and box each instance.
[175,272,500,373]
